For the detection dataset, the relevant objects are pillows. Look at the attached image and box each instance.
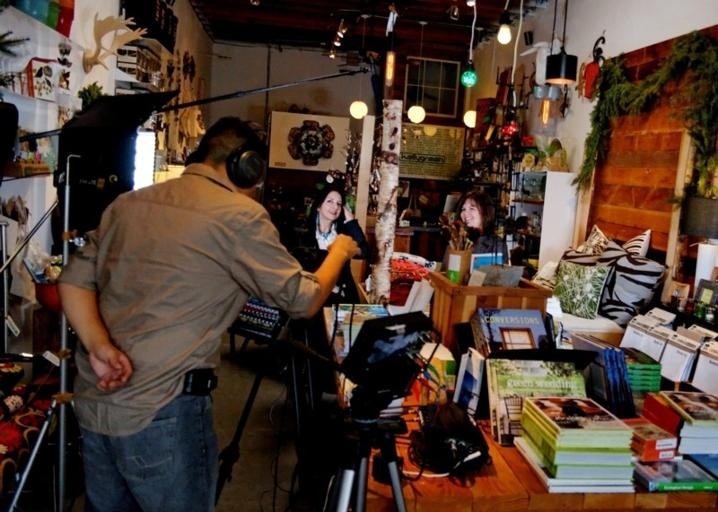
[534,220,670,329]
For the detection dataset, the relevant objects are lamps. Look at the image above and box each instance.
[546,1,580,86]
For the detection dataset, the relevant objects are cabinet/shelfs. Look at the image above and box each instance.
[461,79,528,181]
[505,168,578,269]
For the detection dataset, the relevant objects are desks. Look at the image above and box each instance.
[323,302,717,510]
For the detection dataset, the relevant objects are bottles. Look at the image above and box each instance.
[670,289,715,324]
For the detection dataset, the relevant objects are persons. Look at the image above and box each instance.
[53,115,364,510]
[298,186,371,308]
[458,191,505,257]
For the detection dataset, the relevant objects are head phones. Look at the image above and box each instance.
[183,115,272,191]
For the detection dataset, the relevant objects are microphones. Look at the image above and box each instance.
[48,165,121,194]
[356,61,382,78]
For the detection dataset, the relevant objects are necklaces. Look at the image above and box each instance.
[317,219,332,241]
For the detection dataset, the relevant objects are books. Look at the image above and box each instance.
[334,307,718,492]
[468,252,504,275]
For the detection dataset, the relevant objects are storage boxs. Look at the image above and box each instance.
[428,266,545,348]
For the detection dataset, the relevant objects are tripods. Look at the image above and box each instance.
[6,201,77,512]
[313,418,408,512]
